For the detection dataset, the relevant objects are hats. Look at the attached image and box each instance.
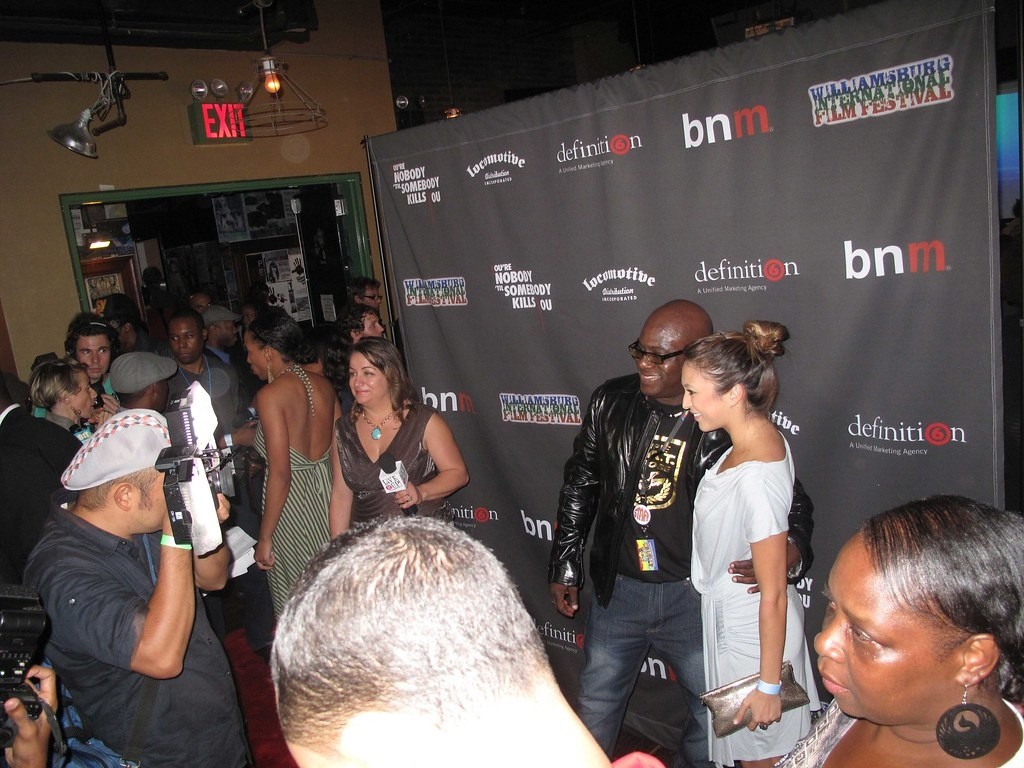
[60,409,170,490]
[110,351,178,392]
[100,294,144,330]
[201,306,240,327]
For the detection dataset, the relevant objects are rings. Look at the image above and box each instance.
[263,563,267,566]
[407,494,412,502]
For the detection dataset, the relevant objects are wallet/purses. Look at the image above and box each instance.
[699,661,809,738]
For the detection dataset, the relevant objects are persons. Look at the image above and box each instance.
[680,318,820,768]
[0,287,268,768]
[544,294,814,768]
[338,273,386,414]
[244,306,342,631]
[774,491,1024,768]
[328,337,470,542]
[268,515,667,768]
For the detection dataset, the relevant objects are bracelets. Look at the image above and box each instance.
[159,534,193,551]
[223,434,233,447]
[757,679,783,695]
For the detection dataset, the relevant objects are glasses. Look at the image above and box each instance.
[629,341,684,365]
[365,295,383,300]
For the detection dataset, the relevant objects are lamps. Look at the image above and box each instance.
[187,79,254,146]
[83,232,111,250]
[230,0,326,135]
[395,95,426,130]
[45,108,96,158]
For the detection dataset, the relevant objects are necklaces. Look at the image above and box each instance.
[363,409,394,441]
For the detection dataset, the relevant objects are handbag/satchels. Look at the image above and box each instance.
[36,659,139,768]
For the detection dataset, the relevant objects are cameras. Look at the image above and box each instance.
[155,407,235,547]
[0,581,47,746]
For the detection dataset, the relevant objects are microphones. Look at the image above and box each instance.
[379,453,418,517]
